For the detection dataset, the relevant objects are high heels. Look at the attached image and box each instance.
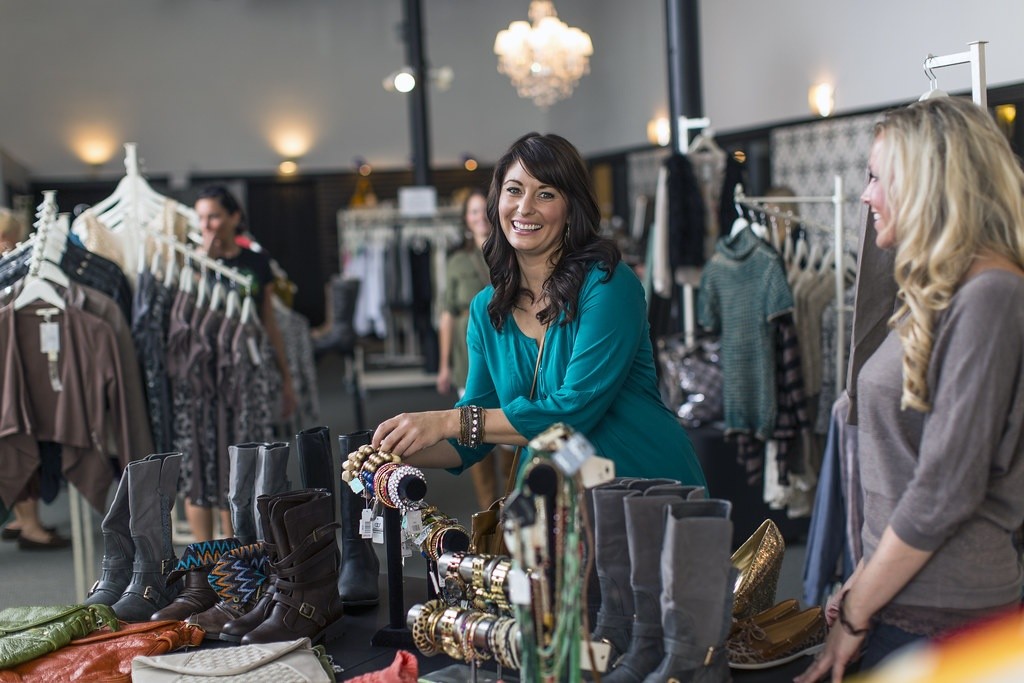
[731,519,786,616]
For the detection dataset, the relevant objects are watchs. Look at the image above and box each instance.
[444,551,473,600]
[442,608,464,660]
[470,553,497,614]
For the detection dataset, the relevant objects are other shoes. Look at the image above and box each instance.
[724,605,832,669]
[731,598,800,645]
[18,532,71,550]
[2,524,56,538]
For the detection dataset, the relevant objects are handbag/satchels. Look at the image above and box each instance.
[0,603,121,669]
[130,637,332,683]
[0,612,206,683]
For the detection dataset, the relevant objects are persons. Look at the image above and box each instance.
[0,209,70,550]
[184,185,275,542]
[793,97,1024,683]
[763,188,809,518]
[372,131,708,487]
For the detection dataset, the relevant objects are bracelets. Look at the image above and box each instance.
[343,443,477,564]
[839,589,869,635]
[413,556,522,670]
[457,405,486,449]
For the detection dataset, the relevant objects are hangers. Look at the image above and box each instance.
[10,199,69,311]
[74,175,204,244]
[137,230,289,328]
[916,58,950,100]
[726,198,858,282]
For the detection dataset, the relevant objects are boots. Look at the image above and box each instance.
[80,426,380,647]
[642,499,735,683]
[599,485,711,683]
[580,476,682,683]
